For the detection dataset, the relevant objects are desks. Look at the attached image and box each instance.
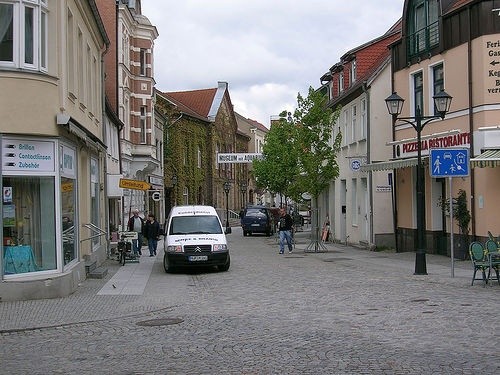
[3,245,35,274]
[487,251,500,286]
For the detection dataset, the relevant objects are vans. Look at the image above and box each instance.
[160,205,232,272]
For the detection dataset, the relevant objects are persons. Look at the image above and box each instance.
[129,210,160,257]
[278,208,292,254]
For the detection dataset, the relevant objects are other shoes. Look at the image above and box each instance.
[138,250,142,255]
[279,252,282,254]
[289,250,292,253]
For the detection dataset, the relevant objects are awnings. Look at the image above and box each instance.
[469,150,500,168]
[359,157,429,176]
[57,114,108,156]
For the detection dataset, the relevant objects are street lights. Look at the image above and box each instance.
[170,175,177,207]
[385,88,454,277]
[240,184,247,210]
[224,182,232,234]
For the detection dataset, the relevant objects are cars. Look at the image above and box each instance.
[242,206,304,238]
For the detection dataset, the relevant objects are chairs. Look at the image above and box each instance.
[469,240,500,287]
